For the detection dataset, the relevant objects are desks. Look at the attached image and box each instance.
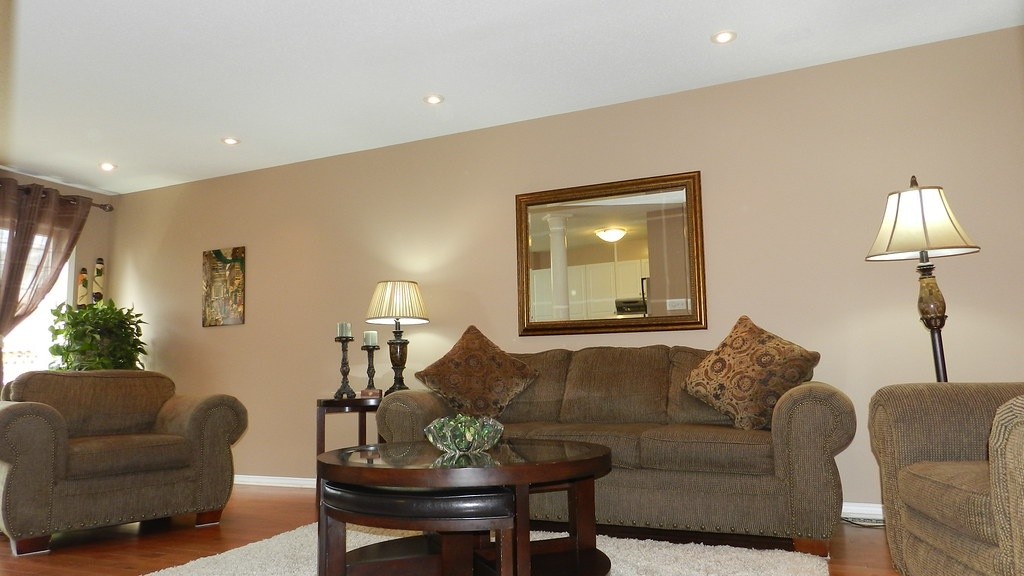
[314,397,381,511]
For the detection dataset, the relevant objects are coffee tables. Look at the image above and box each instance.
[318,434,613,576]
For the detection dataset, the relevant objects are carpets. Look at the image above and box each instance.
[142,520,829,576]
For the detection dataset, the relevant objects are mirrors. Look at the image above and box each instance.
[516,170,707,337]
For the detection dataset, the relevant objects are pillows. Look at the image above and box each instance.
[668,347,734,426]
[680,315,820,428]
[559,344,671,423]
[415,326,539,418]
[12,371,175,438]
[501,351,577,422]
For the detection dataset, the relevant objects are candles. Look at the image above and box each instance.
[337,321,352,337]
[364,331,378,345]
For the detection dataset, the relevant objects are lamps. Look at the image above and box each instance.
[365,281,430,395]
[866,176,981,382]
[594,226,627,242]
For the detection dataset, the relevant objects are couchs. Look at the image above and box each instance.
[868,382,1024,576]
[375,343,856,554]
[0,370,248,556]
